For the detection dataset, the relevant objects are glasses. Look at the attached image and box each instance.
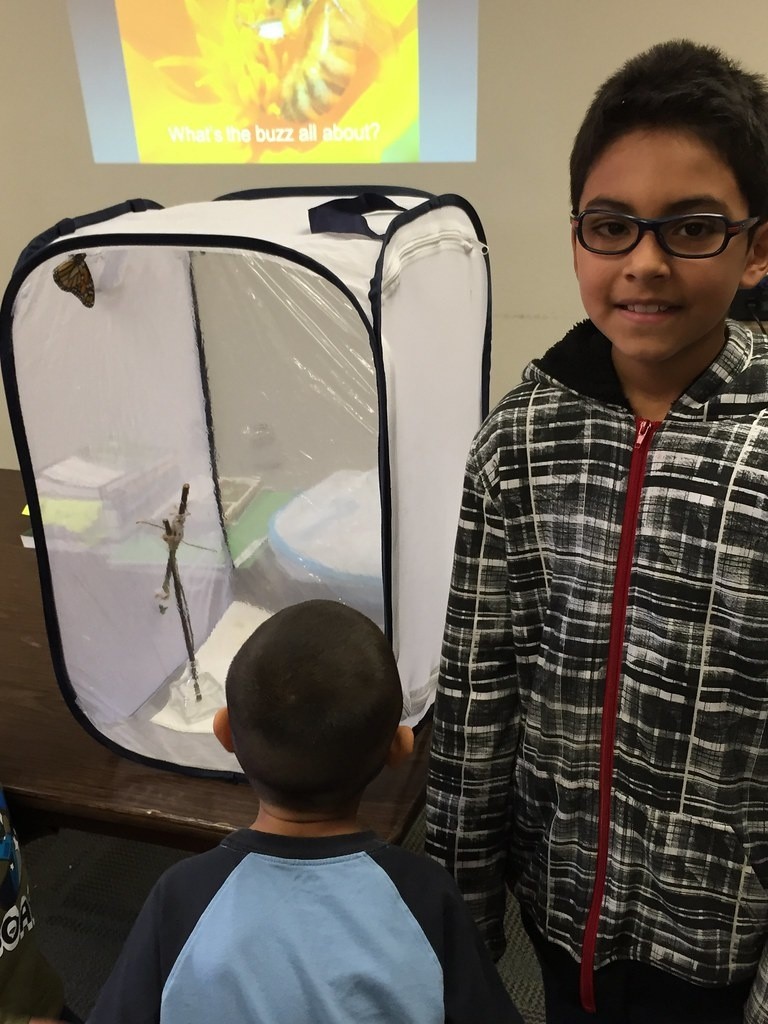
[569,209,763,259]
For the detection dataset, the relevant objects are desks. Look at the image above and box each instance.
[1,467,425,854]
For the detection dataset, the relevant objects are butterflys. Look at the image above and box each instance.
[53,253,95,308]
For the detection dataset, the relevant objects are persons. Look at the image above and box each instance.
[424,40,768,1024]
[86,600,517,1023]
